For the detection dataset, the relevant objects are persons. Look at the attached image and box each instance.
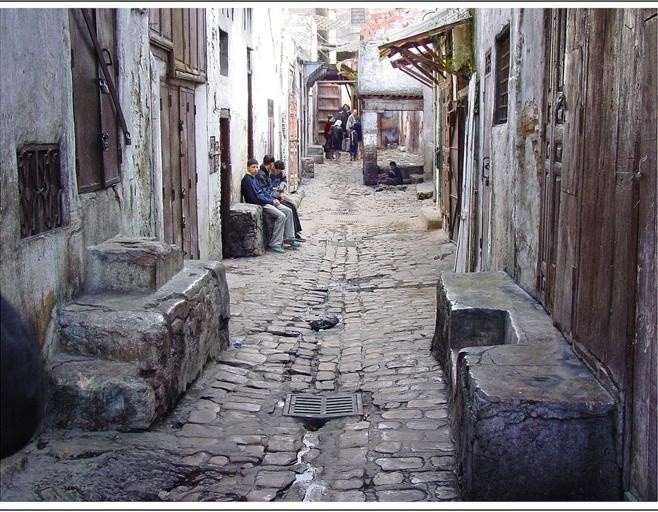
[323,104,366,161]
[380,160,403,184]
[240,155,306,254]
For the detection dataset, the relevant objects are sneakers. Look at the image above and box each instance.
[266,233,306,253]
